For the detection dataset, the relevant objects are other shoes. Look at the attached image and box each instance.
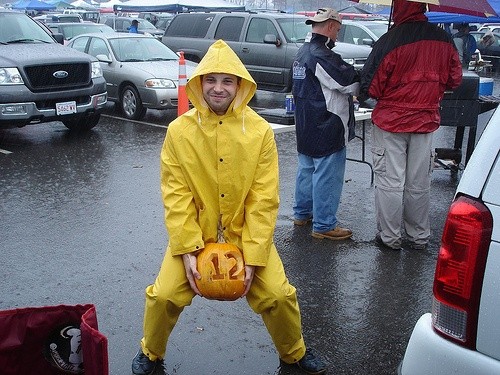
[280,351,327,375]
[132,349,158,375]
[411,244,428,250]
[376,235,401,249]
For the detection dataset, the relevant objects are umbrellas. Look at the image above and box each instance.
[348,0,497,19]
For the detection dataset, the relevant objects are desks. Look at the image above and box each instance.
[268,108,374,186]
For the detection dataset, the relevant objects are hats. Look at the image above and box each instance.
[305,8,342,26]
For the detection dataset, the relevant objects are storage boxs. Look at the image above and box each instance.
[479,77,494,96]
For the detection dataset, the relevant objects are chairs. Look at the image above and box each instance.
[475,51,494,77]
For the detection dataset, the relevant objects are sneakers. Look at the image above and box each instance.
[312,227,354,240]
[294,216,313,225]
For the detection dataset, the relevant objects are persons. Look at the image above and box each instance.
[126,20,140,33]
[131,39,327,375]
[361,0,477,249]
[293,8,363,240]
[150,16,158,24]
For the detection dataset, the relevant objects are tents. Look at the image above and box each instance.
[6,0,246,33]
[375,0,500,23]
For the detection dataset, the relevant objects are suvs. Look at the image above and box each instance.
[0,7,111,133]
[162,10,376,105]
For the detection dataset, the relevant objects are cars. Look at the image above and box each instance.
[298,8,500,71]
[330,20,395,48]
[392,104,500,374]
[67,32,200,120]
[27,8,173,45]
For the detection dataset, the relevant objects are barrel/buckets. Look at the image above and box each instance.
[285,95,295,114]
[479,76,493,97]
